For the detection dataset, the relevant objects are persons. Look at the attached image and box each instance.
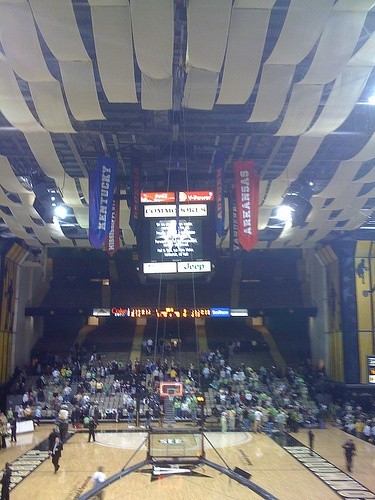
[342,438,357,472]
[0,463,14,500]
[308,430,317,456]
[0,412,17,449]
[8,338,375,444]
[87,417,97,443]
[48,428,63,474]
[92,466,108,500]
